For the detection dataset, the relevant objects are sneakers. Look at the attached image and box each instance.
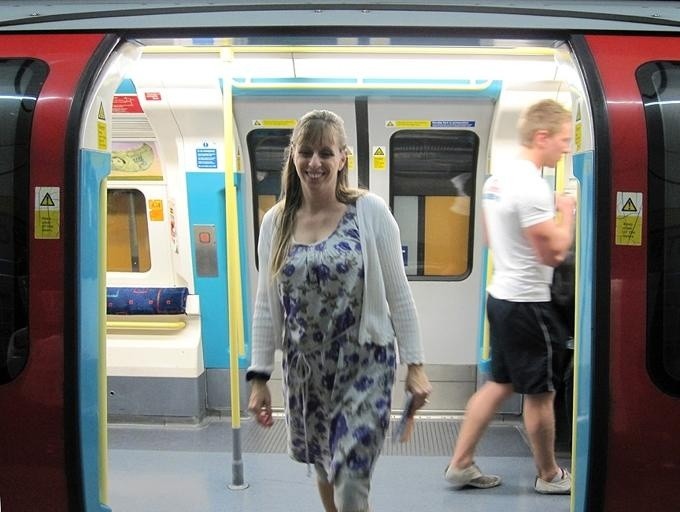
[535,467,571,495]
[445,461,501,488]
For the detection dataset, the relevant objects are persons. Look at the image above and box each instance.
[441,99,577,495]
[245,109,434,511]
